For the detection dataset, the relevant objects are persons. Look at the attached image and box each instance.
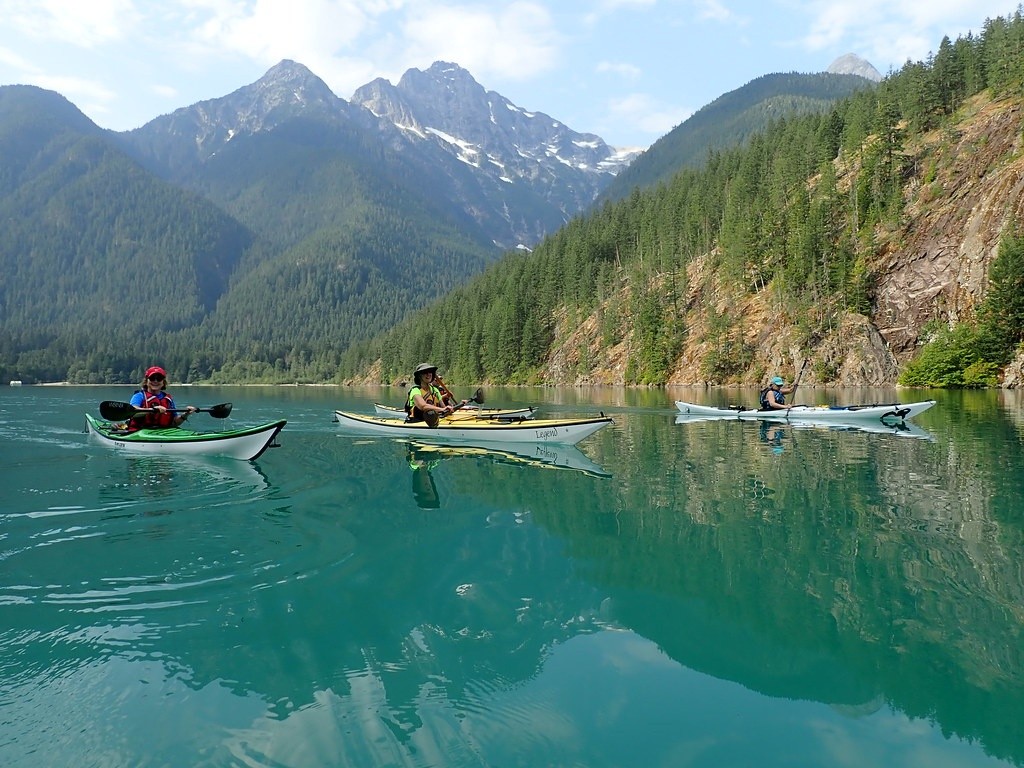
[765,377,797,410]
[405,362,467,422]
[760,422,786,455]
[124,366,195,433]
[406,451,444,510]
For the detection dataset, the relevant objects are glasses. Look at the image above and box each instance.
[776,385,782,387]
[147,376,164,382]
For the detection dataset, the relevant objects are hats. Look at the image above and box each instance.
[772,377,783,386]
[145,366,166,378]
[414,364,438,385]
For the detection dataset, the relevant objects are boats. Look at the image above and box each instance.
[83,412,288,461]
[392,437,614,483]
[372,402,540,421]
[334,409,613,452]
[673,400,938,428]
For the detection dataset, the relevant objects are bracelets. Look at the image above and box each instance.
[181,413,189,419]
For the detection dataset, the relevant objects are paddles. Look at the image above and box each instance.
[436,374,459,405]
[100,400,232,421]
[426,387,484,426]
